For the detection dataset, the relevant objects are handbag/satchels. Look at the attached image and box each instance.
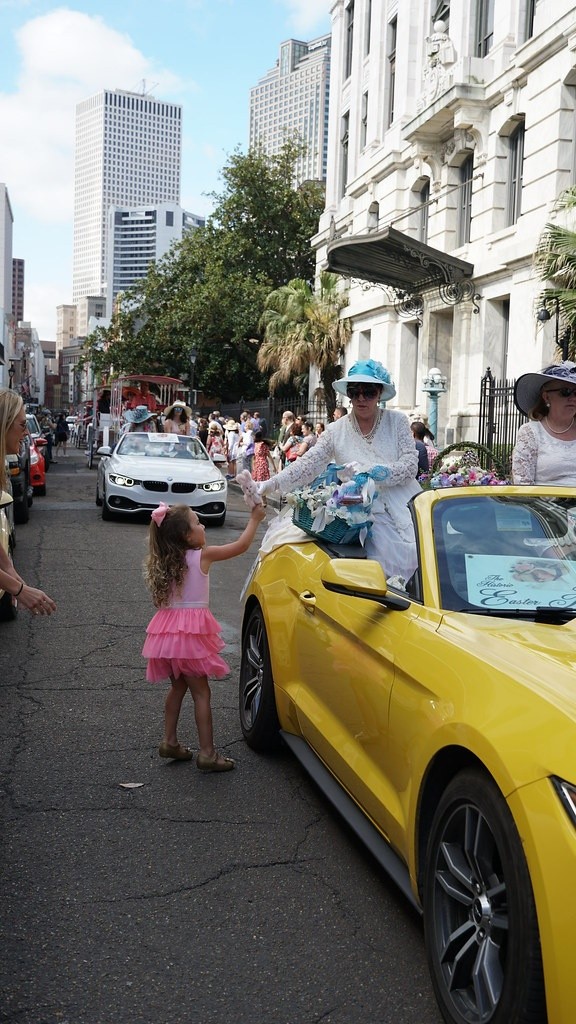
[65,431,71,439]
[285,437,303,461]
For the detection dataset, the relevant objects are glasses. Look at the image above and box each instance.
[9,419,28,431]
[347,383,377,399]
[542,387,576,397]
[172,407,183,412]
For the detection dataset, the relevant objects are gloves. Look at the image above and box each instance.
[342,461,368,476]
[255,479,276,497]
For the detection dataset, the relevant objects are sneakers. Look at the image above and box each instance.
[225,473,235,482]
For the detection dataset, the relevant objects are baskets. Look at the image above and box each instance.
[286,465,376,545]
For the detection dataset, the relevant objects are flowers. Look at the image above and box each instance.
[289,477,356,522]
[417,462,511,490]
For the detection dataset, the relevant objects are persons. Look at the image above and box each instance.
[272,411,324,504]
[190,411,278,508]
[410,422,443,480]
[38,389,111,463]
[333,407,347,422]
[0,388,56,616]
[141,501,266,772]
[513,361,576,561]
[122,382,201,457]
[257,365,462,585]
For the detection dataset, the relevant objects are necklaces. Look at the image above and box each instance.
[545,416,574,433]
[350,408,381,442]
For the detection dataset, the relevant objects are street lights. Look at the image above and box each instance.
[6,363,17,392]
[421,368,449,447]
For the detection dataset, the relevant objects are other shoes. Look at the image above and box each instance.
[158,742,193,760]
[197,750,235,772]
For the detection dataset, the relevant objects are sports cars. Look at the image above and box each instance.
[94,432,230,526]
[237,484,575,1024]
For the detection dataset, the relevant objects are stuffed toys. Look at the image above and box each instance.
[236,471,266,521]
[306,468,358,510]
[431,464,501,486]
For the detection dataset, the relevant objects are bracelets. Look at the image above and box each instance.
[13,583,23,596]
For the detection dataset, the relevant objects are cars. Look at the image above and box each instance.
[0,403,58,622]
[65,416,77,433]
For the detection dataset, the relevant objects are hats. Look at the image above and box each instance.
[513,360,576,418]
[44,409,51,415]
[122,387,141,399]
[332,360,396,402]
[164,400,192,417]
[410,414,430,429]
[144,391,156,400]
[124,406,157,423]
[223,419,238,431]
